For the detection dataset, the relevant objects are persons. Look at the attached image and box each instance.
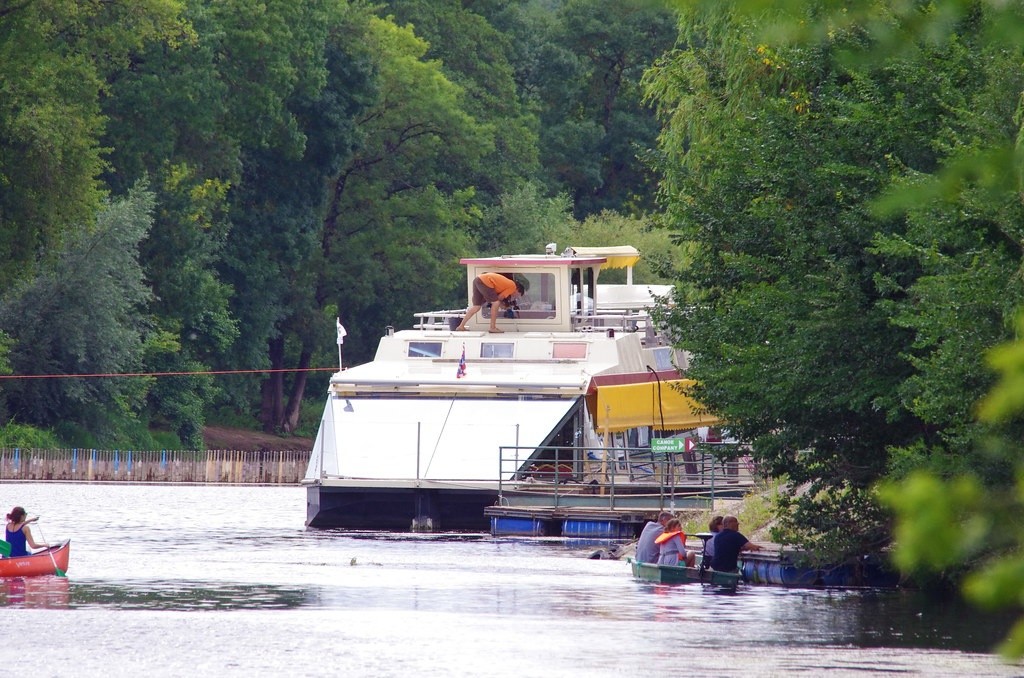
[511,272,529,310]
[455,273,524,334]
[635,512,695,568]
[2,507,49,558]
[705,515,761,575]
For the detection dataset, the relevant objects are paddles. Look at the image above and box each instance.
[0,539,12,558]
[35,519,66,577]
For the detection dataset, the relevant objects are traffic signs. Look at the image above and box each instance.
[650,438,696,453]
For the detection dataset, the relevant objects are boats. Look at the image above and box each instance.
[299,240,732,532]
[0,538,71,577]
[624,531,745,588]
